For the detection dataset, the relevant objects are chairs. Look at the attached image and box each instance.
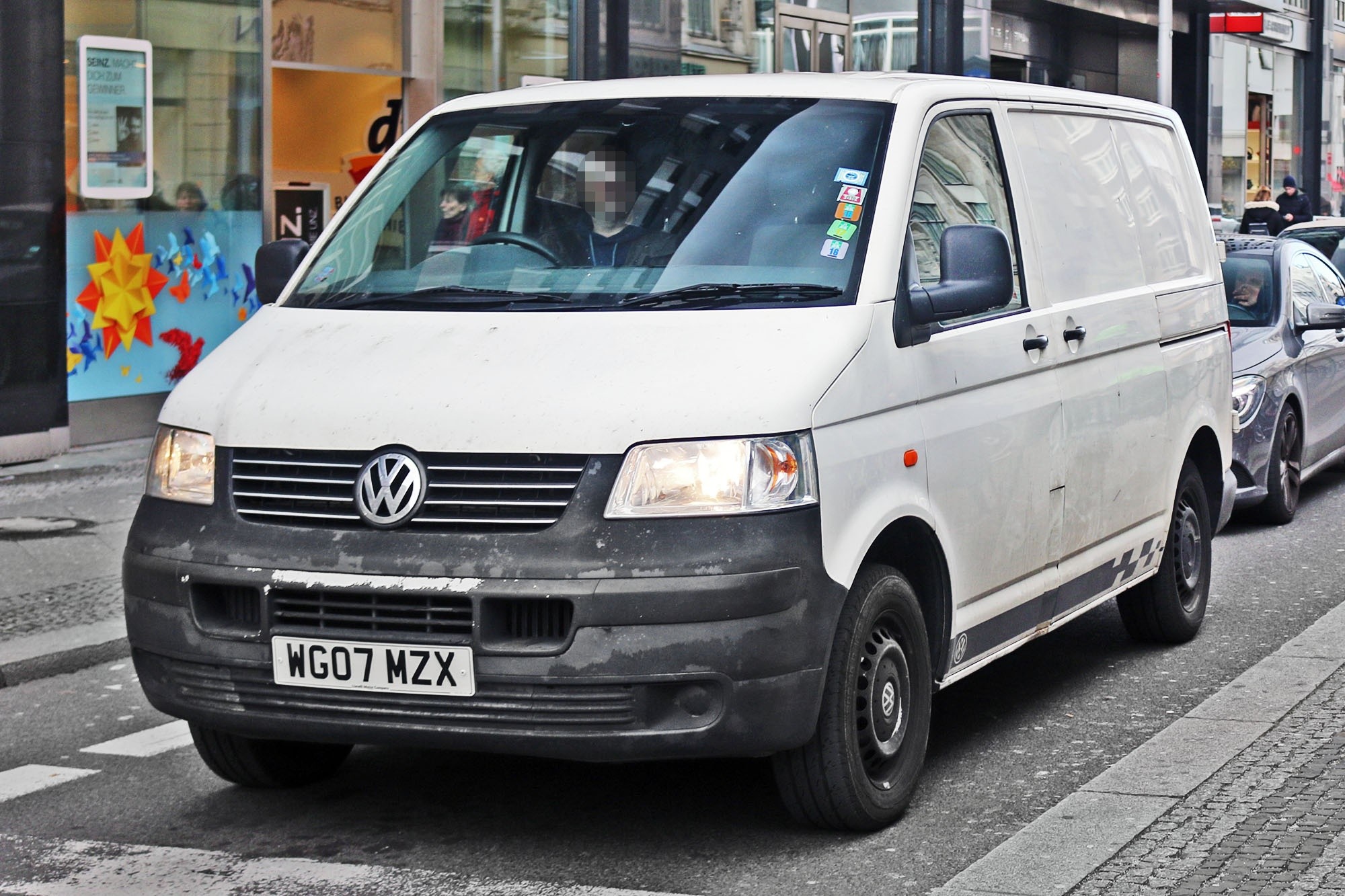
[748,148,859,267]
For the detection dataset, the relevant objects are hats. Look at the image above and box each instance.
[1282,176,1297,189]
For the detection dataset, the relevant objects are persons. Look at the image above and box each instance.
[176,183,229,257]
[118,113,143,167]
[1276,176,1313,227]
[1225,262,1272,320]
[465,149,509,243]
[430,185,473,257]
[1239,185,1283,237]
[485,152,653,267]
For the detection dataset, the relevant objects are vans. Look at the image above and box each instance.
[122,72,1239,833]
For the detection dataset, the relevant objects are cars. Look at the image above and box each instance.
[1215,232,1345,525]
[1278,218,1345,280]
